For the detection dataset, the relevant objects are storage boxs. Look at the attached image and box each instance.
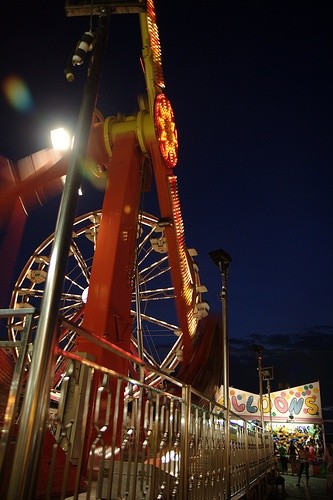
[95,459,176,500]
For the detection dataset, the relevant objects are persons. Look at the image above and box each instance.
[270,438,333,484]
